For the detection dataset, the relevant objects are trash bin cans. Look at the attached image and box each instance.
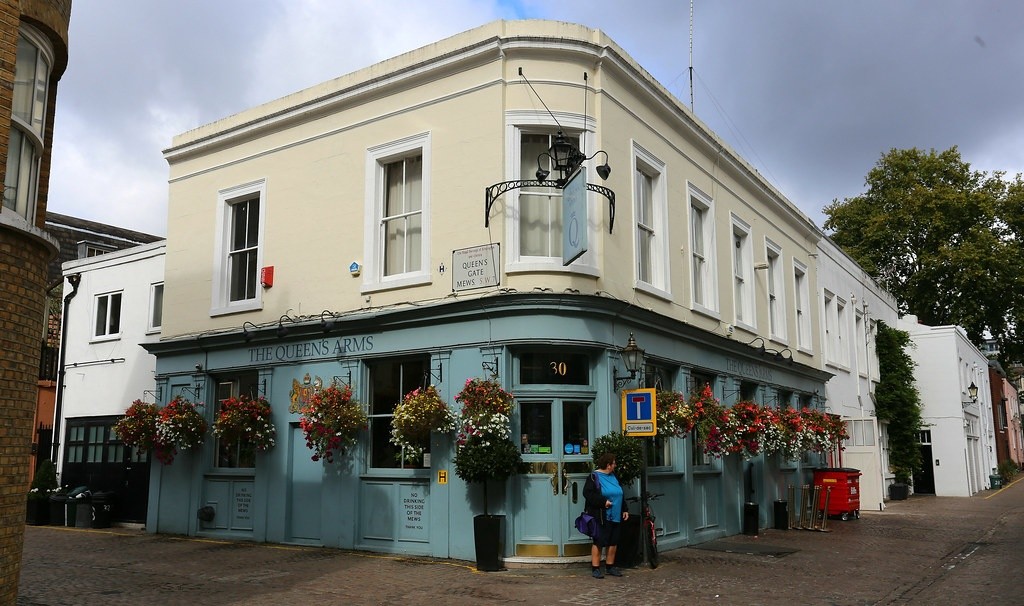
[988,475,1001,488]
[814,468,861,521]
[26,487,112,529]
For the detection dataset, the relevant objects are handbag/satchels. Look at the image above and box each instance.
[574,512,599,538]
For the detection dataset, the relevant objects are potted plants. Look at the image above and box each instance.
[591,429,649,571]
[451,433,523,573]
[870,318,936,502]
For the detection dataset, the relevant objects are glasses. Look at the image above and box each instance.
[610,463,617,465]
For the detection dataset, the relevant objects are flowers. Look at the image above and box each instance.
[453,377,515,446]
[111,389,209,467]
[653,382,850,468]
[299,377,370,464]
[208,389,278,466]
[387,383,457,465]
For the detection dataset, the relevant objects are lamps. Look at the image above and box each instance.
[968,381,979,404]
[765,349,786,363]
[536,129,611,183]
[780,348,794,366]
[619,332,646,378]
[242,321,259,344]
[743,333,766,355]
[319,309,338,334]
[276,316,294,340]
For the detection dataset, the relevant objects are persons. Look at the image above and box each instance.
[574,453,629,578]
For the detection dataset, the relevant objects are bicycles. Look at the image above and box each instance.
[624,491,666,569]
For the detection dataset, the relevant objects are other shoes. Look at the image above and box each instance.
[605,568,622,577]
[591,568,604,578]
